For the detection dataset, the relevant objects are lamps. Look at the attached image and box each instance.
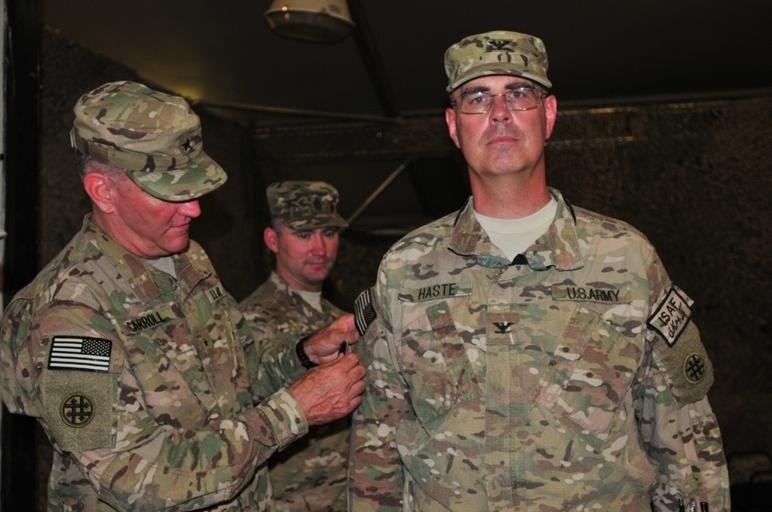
[264,0,356,46]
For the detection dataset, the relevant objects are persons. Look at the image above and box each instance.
[347,31,731,511]
[0,80,367,511]
[237,181,354,511]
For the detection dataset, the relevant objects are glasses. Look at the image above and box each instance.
[451,86,547,115]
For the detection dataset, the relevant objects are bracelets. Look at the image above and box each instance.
[296,337,319,369]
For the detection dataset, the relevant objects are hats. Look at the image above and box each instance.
[265,178,350,232]
[444,29,553,93]
[69,79,231,204]
